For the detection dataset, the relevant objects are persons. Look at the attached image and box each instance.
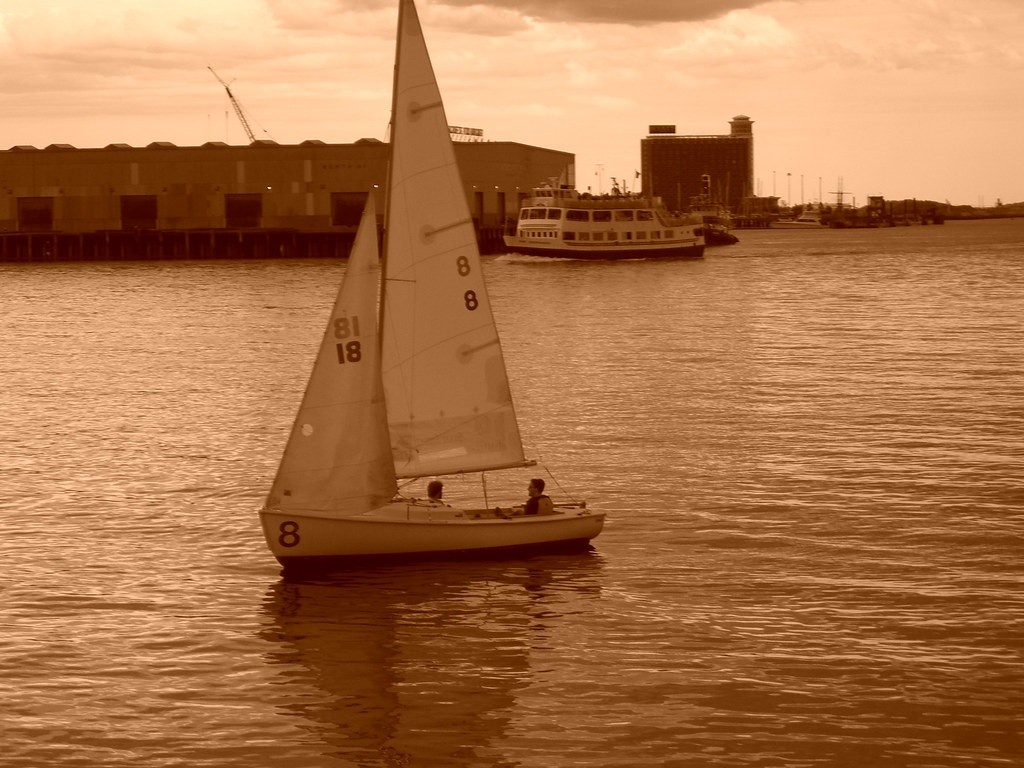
[426,480,444,503]
[526,479,554,515]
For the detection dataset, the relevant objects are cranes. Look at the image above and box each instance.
[208,66,276,142]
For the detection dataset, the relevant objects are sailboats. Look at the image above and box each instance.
[257,0,606,574]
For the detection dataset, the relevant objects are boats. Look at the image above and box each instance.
[730,178,778,227]
[681,174,730,244]
[503,159,705,259]
[822,197,882,228]
